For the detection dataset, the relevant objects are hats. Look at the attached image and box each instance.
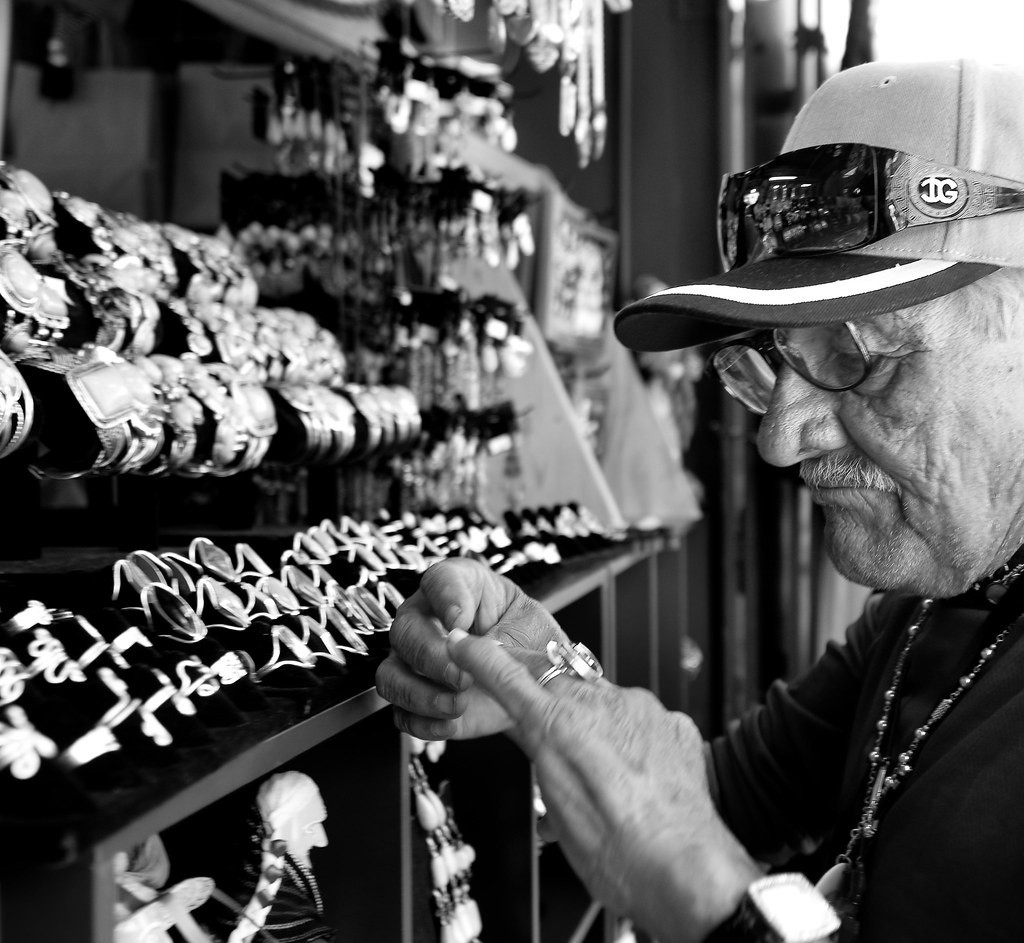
[613,54,1023,353]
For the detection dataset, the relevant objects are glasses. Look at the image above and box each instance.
[703,322,876,414]
[716,143,1023,272]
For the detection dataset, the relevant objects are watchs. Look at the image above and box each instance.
[704,873,841,943]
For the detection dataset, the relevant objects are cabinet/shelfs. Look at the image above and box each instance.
[0,501,690,943]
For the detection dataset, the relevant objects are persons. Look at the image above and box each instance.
[375,45,1024,943]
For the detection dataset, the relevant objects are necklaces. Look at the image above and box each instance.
[815,563,1024,902]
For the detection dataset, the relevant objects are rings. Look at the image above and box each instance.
[0,159,423,477]
[538,641,602,691]
[0,504,582,779]
